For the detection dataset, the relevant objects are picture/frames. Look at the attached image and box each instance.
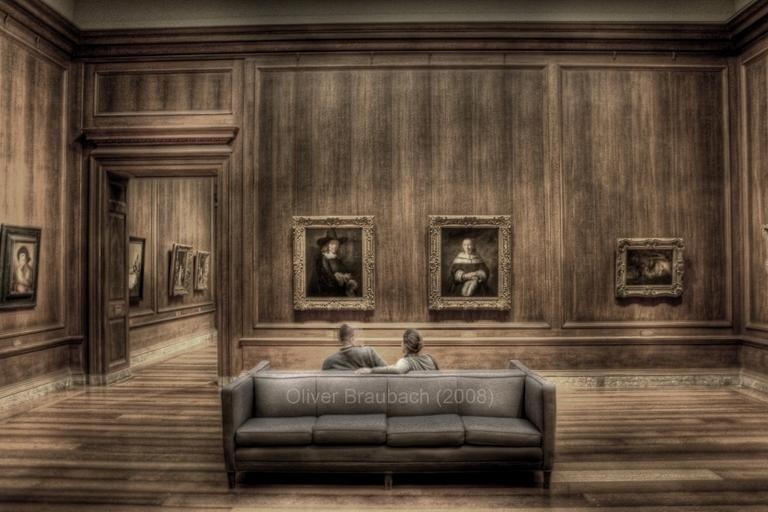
[0,224,41,310]
[615,237,685,299]
[290,213,513,311]
[129,235,211,302]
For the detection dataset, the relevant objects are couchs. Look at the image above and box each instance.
[220,358,556,490]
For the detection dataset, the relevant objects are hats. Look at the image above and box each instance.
[317,229,348,246]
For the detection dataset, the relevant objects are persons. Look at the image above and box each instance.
[11,245,33,295]
[447,235,496,296]
[321,322,388,369]
[354,328,440,374]
[308,228,361,297]
